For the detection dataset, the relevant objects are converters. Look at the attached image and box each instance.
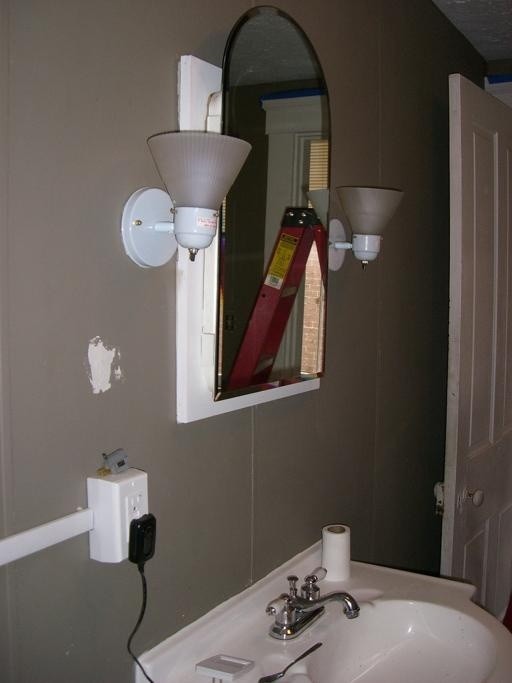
[129,513,156,563]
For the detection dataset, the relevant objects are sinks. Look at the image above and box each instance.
[134,536,512,683]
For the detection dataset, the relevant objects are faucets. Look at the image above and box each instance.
[267,574,361,639]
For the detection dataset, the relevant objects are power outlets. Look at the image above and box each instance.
[86,470,149,566]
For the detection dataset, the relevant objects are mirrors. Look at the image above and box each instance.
[215,2,334,402]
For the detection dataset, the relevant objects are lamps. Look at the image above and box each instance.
[329,184,406,275]
[118,127,256,271]
